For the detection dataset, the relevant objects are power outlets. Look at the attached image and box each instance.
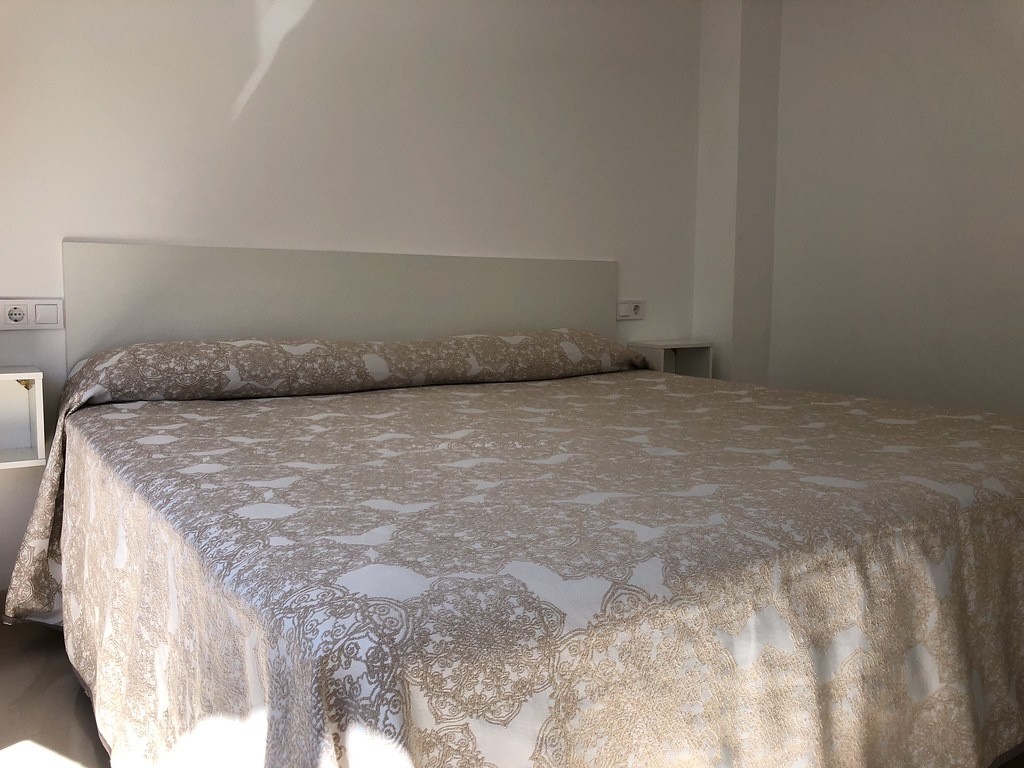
[616,299,644,320]
[0,299,63,331]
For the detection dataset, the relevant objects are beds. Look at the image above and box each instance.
[0,235,1024,768]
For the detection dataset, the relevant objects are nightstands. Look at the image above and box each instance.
[0,365,48,470]
[628,338,714,379]
